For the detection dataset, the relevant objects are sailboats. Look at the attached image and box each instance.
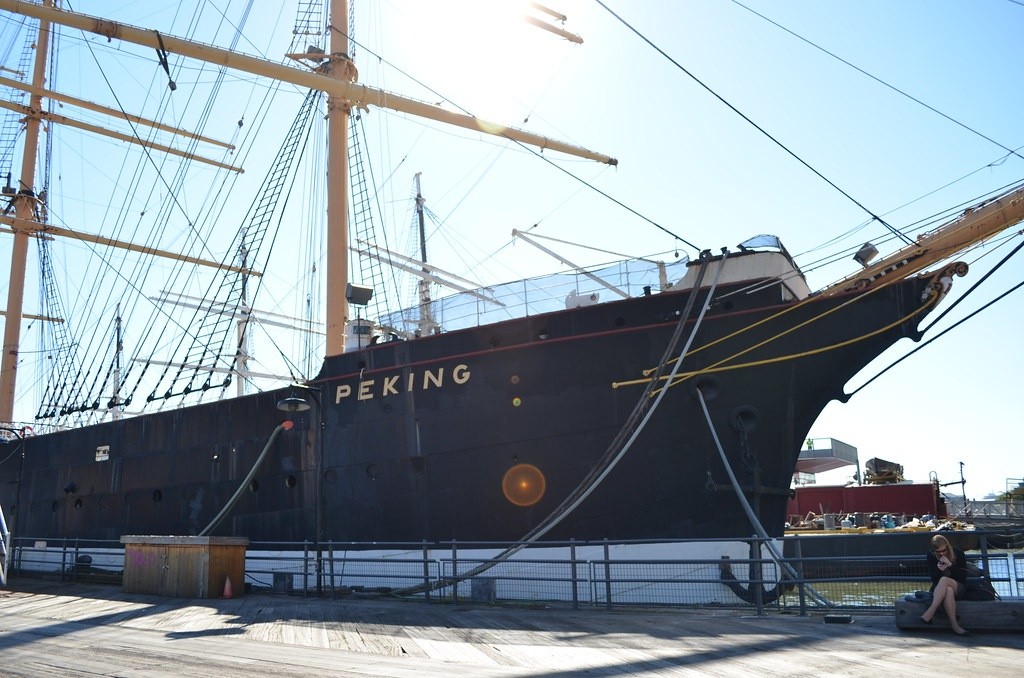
[0,1,1024,606]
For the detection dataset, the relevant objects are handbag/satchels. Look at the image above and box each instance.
[978,579,1002,601]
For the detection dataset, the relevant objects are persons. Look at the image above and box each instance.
[853,471,858,480]
[863,472,867,484]
[806,438,813,450]
[919,535,970,636]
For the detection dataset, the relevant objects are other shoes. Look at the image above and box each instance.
[952,627,970,636]
[920,617,933,625]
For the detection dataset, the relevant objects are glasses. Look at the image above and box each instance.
[935,547,948,553]
